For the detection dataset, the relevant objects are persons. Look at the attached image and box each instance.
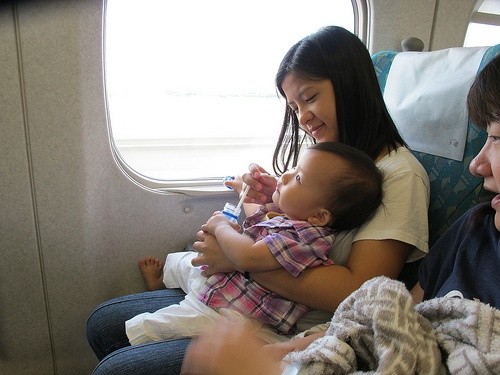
[81,25,431,375]
[123,140,386,351]
[180,49,499,375]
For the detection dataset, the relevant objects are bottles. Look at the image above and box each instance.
[195,202,241,271]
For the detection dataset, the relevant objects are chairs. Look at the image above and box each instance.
[371,44,500,291]
